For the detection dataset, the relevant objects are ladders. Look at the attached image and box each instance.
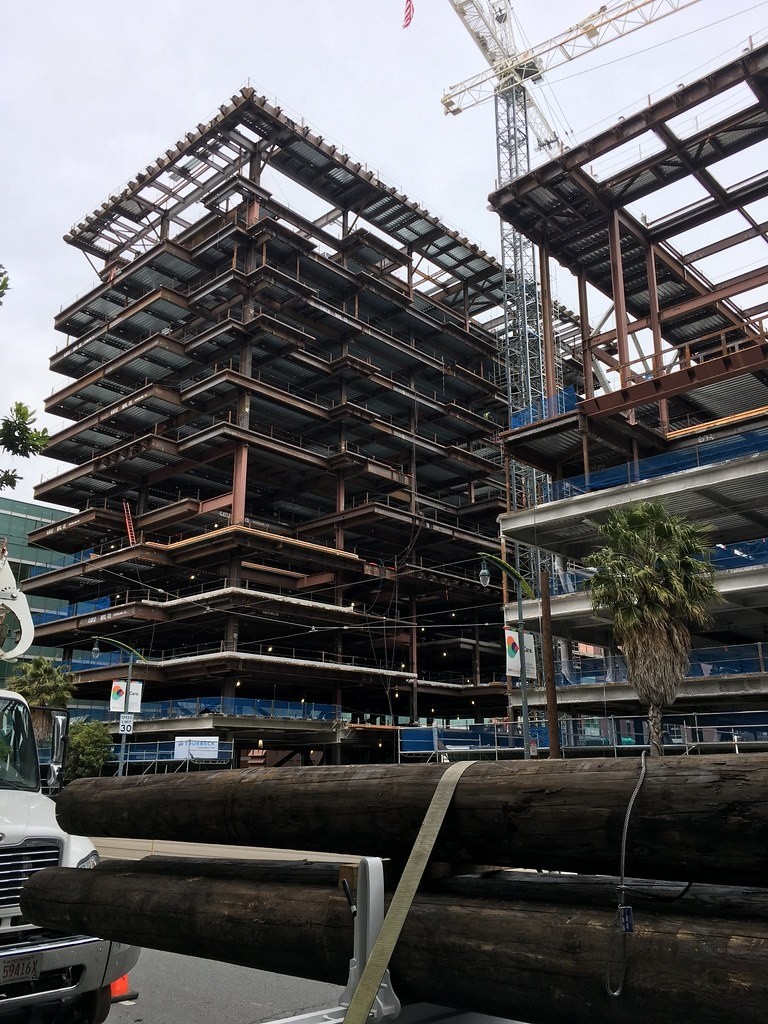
[121,498,137,546]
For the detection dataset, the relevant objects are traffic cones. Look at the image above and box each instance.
[108,973,139,1003]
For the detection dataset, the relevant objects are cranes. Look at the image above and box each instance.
[439,0,767,758]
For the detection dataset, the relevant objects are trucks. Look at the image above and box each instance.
[0,689,141,1024]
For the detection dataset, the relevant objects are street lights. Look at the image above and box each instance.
[476,552,537,758]
[92,635,148,776]
